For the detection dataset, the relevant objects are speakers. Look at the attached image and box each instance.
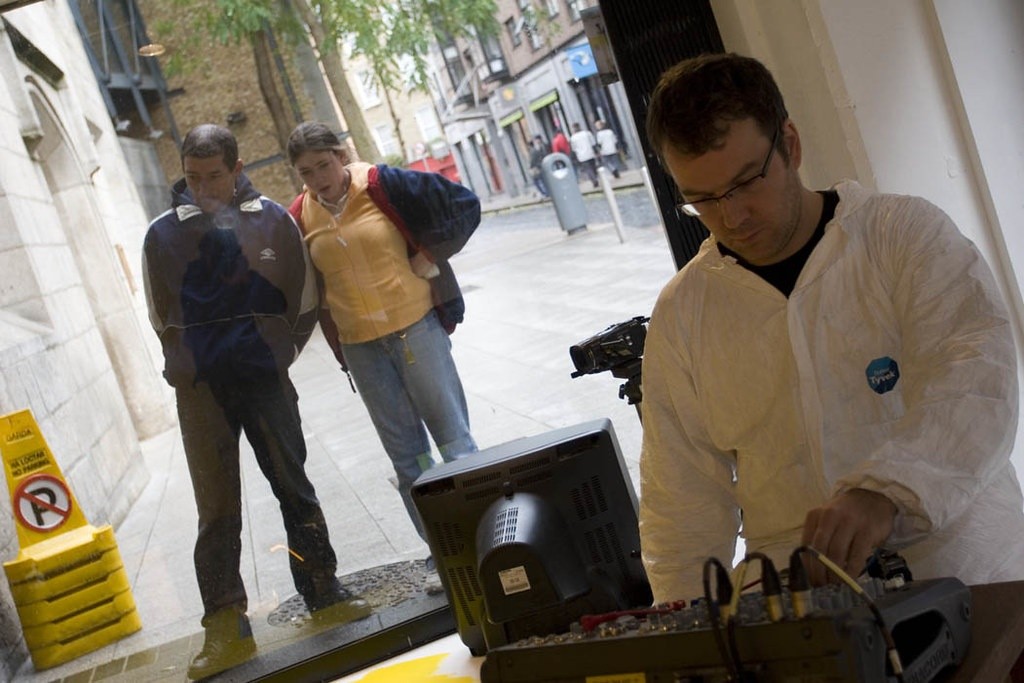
[408,419,657,658]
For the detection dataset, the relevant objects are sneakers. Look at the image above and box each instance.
[304,584,374,623]
[187,609,256,681]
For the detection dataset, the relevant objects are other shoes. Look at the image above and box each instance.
[423,558,442,592]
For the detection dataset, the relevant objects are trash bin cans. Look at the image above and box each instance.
[540,152,588,235]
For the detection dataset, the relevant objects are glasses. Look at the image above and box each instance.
[672,125,781,216]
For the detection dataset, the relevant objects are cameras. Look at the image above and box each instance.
[567,315,650,380]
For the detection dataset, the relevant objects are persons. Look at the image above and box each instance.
[528,119,624,201]
[143,125,372,678]
[288,121,490,593]
[640,51,1024,610]
